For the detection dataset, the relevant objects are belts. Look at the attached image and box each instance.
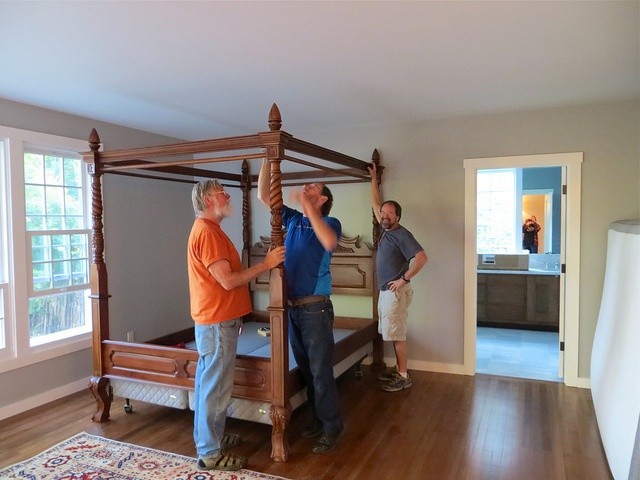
[289,295,329,305]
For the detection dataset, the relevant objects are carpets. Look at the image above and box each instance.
[0,431,289,480]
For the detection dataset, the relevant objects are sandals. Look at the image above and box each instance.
[197,453,246,471]
[220,433,241,448]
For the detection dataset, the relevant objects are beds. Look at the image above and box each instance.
[76,101,385,464]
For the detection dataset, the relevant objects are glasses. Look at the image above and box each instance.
[301,183,324,192]
[210,190,226,196]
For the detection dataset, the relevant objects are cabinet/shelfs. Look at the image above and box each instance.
[477,274,525,323]
[525,275,559,325]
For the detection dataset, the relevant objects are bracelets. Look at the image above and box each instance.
[401,275,411,283]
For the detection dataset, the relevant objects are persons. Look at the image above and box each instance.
[256,157,346,454]
[522,216,542,254]
[187,179,286,471]
[367,161,428,393]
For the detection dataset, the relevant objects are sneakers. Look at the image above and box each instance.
[382,371,413,391]
[312,421,346,453]
[376,369,395,380]
[302,420,322,438]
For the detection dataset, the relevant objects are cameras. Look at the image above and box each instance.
[528,224,534,228]
